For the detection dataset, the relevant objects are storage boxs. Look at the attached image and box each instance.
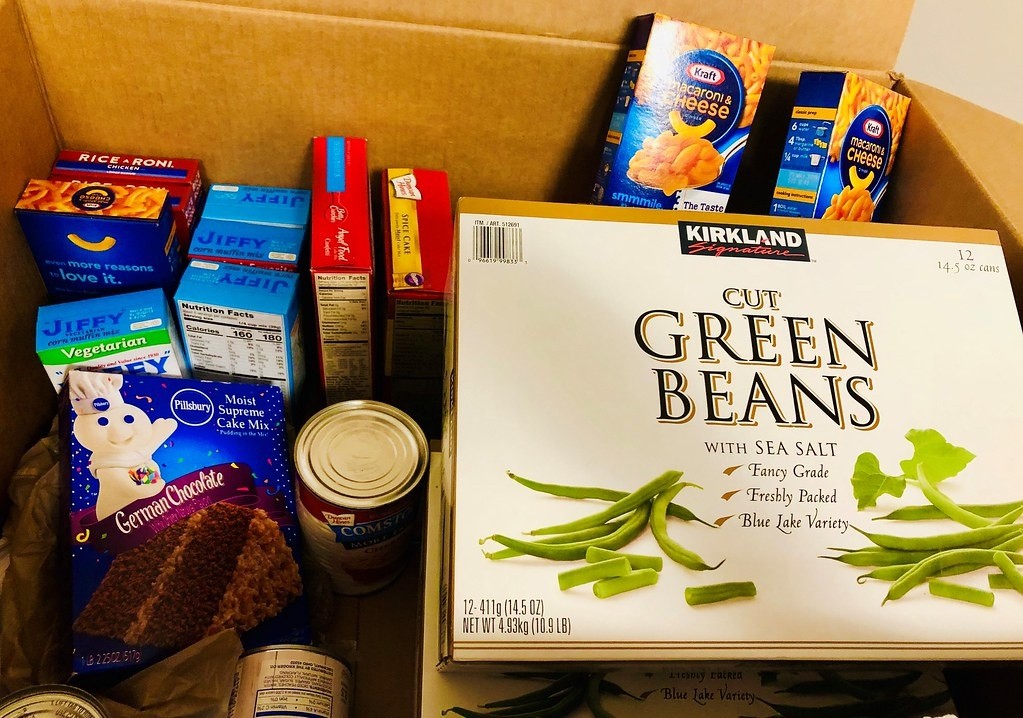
[0,0,1022,718]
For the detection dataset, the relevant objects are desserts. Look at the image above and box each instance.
[74,505,301,648]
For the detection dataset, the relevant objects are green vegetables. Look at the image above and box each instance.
[852,428,977,512]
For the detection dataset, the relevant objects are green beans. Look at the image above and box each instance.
[816,463,1023,607]
[480,468,758,605]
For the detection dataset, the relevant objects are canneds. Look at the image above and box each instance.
[224,643,354,718]
[291,399,431,595]
[0,682,111,718]
